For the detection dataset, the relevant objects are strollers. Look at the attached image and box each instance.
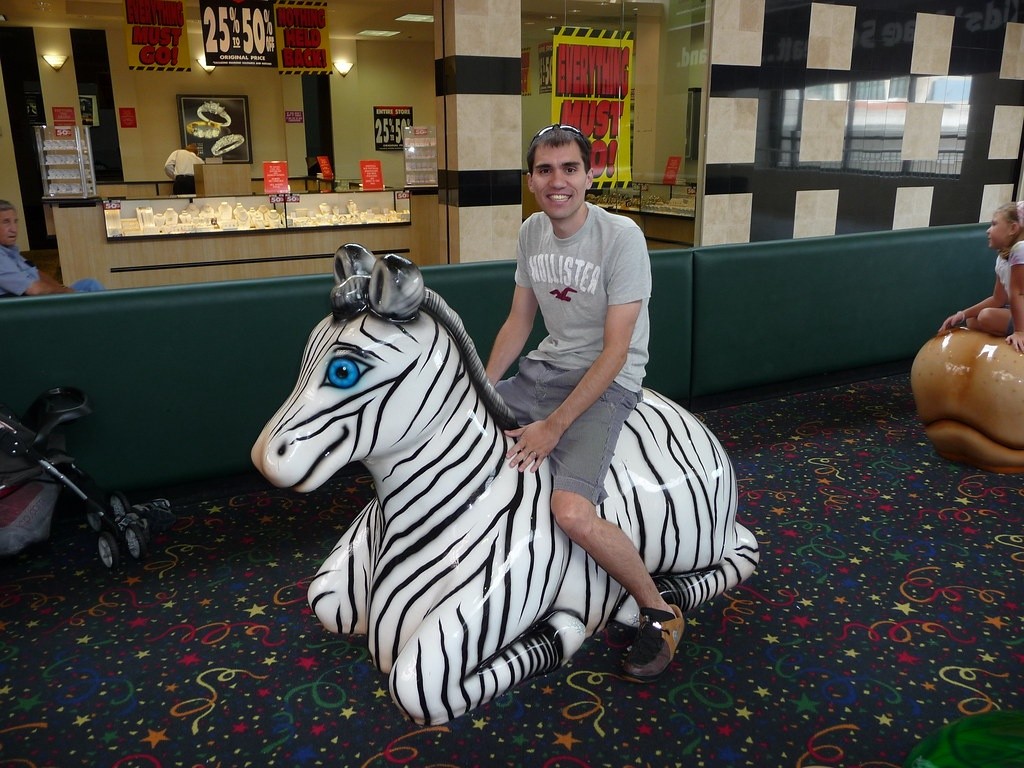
[1,385,146,569]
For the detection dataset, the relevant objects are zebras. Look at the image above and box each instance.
[251,242,759,726]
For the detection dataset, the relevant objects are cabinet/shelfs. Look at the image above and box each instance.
[34,126,96,199]
[403,126,438,188]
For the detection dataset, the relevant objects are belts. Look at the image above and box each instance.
[176,175,187,177]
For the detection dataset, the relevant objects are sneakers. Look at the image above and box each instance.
[622,604,684,682]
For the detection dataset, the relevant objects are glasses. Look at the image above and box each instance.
[530,124,590,148]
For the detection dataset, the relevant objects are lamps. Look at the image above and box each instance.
[42,55,69,71]
[195,59,216,75]
[333,62,354,77]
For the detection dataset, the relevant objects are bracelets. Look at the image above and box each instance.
[956,311,965,324]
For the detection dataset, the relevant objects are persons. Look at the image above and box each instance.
[164,143,205,195]
[936,199,1024,355]
[0,199,108,299]
[482,124,686,678]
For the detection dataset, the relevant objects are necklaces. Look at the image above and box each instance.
[140,208,280,230]
